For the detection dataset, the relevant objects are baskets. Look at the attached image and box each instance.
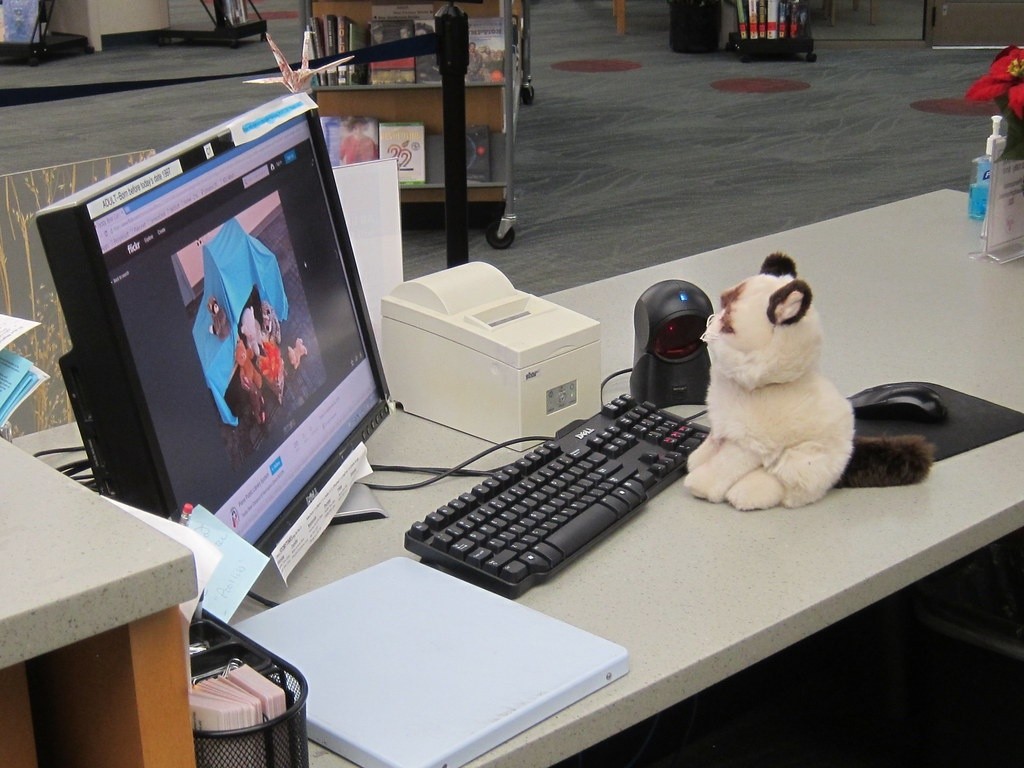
[189,605,308,768]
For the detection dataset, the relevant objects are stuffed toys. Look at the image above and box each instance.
[235,302,285,424]
[205,297,231,339]
[684,252,935,511]
[289,339,307,370]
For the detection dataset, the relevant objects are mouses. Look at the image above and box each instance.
[849,384,947,422]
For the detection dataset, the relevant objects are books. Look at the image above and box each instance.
[306,16,507,185]
[224,0,246,24]
[736,0,808,40]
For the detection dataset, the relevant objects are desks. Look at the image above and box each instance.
[0,181,1024,768]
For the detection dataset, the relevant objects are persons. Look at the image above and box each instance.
[340,116,377,164]
[399,25,411,39]
[373,29,383,45]
[414,22,442,82]
[466,43,485,81]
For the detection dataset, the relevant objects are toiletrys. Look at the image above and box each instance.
[966,114,1005,220]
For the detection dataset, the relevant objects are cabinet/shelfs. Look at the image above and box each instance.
[301,0,536,250]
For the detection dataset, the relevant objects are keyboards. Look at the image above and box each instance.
[404,393,712,600]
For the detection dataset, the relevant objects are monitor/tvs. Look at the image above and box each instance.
[33,92,396,602]
